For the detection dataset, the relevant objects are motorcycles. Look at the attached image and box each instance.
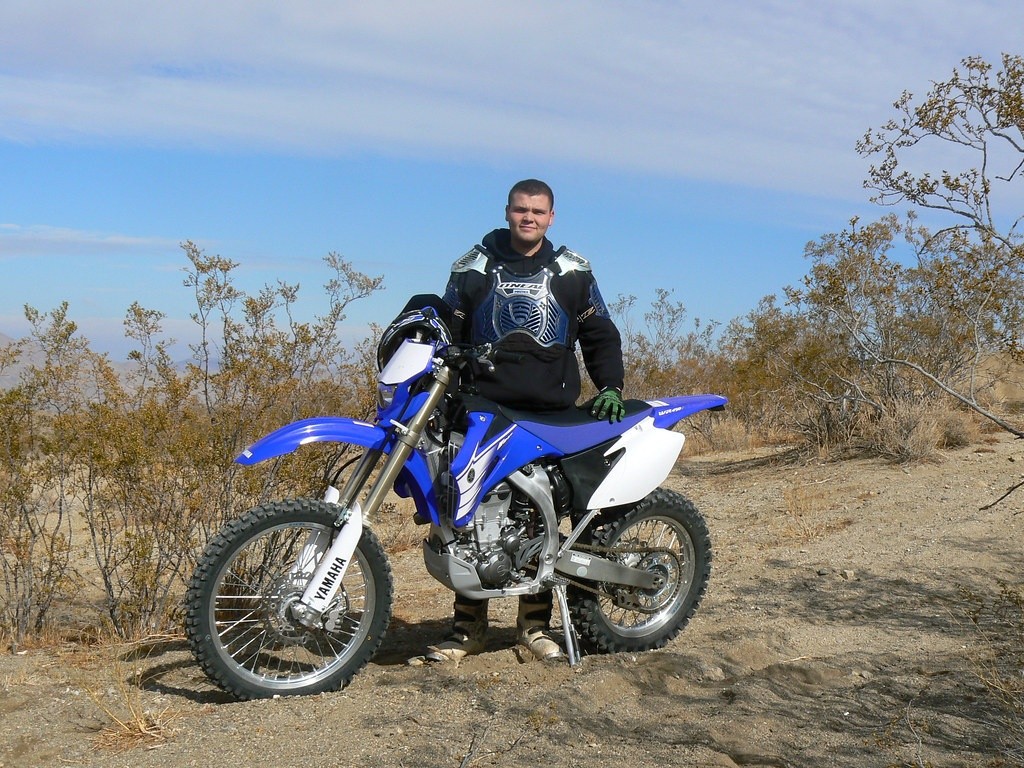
[180,306,731,703]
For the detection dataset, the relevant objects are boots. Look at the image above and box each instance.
[426,603,488,660]
[517,601,563,661]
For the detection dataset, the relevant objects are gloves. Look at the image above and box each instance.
[584,386,625,423]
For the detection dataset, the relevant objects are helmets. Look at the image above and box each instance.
[376,307,453,394]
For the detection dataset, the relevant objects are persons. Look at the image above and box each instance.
[425,179,625,663]
